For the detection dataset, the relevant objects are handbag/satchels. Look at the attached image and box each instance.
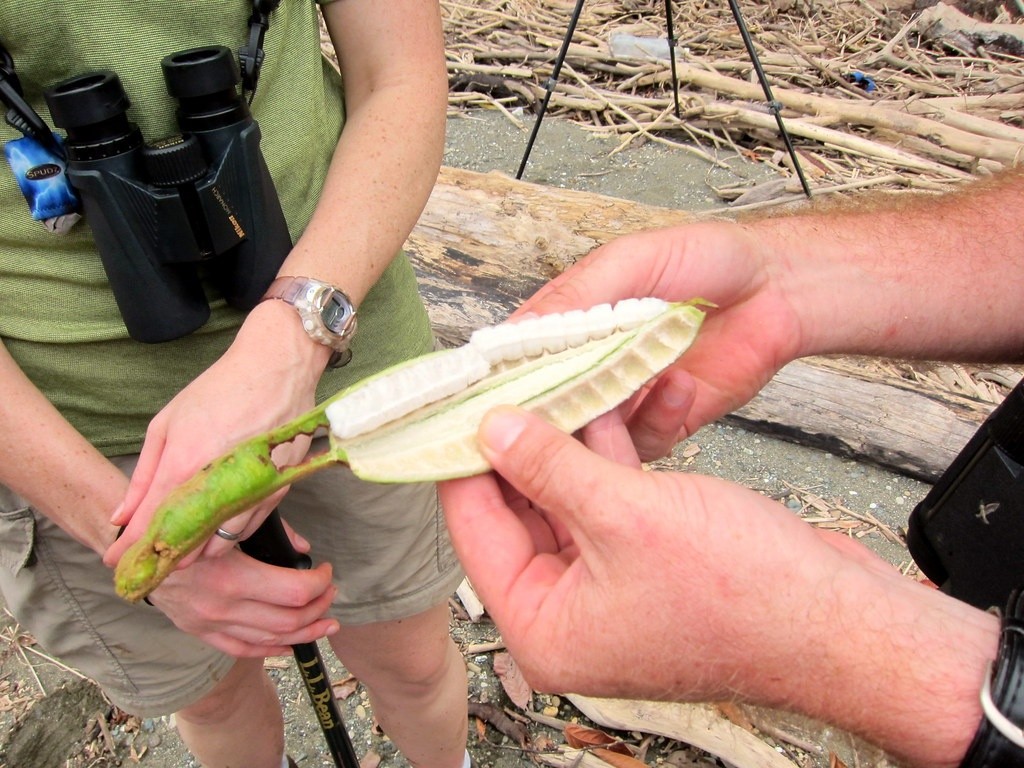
[906,378,1024,616]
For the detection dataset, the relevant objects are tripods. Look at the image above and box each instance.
[515,1,813,201]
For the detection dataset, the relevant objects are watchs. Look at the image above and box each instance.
[261,276,357,368]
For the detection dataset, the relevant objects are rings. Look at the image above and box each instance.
[215,527,245,540]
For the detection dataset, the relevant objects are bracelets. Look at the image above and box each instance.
[958,587,1024,768]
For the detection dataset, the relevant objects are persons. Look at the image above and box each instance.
[0,0,470,768]
[438,166,1024,768]
